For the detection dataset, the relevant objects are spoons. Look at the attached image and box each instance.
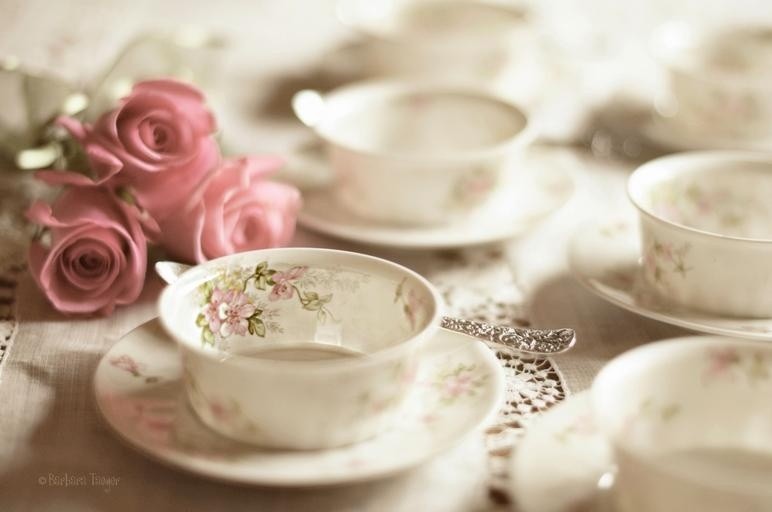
[153,263,574,358]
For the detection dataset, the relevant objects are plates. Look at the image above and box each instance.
[568,209,771,339]
[506,392,608,510]
[93,308,508,489]
[263,138,547,247]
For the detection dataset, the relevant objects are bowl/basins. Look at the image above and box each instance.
[345,3,771,142]
[156,248,443,453]
[592,335,770,512]
[295,77,530,225]
[635,151,771,315]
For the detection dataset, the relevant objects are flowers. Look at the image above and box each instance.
[21,76,304,320]
